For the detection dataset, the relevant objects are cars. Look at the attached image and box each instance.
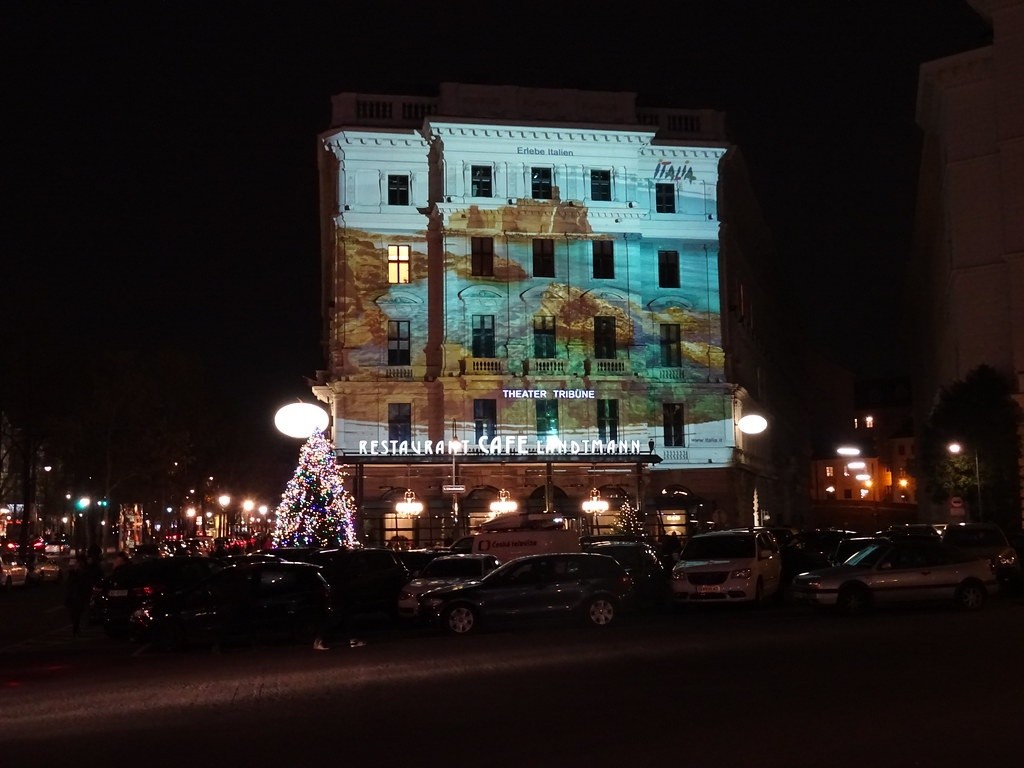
[395,552,503,619]
[588,539,665,617]
[785,520,1020,604]
[96,535,402,643]
[796,530,1000,612]
[669,525,786,609]
[0,542,77,586]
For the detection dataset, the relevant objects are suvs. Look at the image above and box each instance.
[418,550,636,630]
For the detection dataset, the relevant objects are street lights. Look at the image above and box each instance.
[947,441,984,521]
